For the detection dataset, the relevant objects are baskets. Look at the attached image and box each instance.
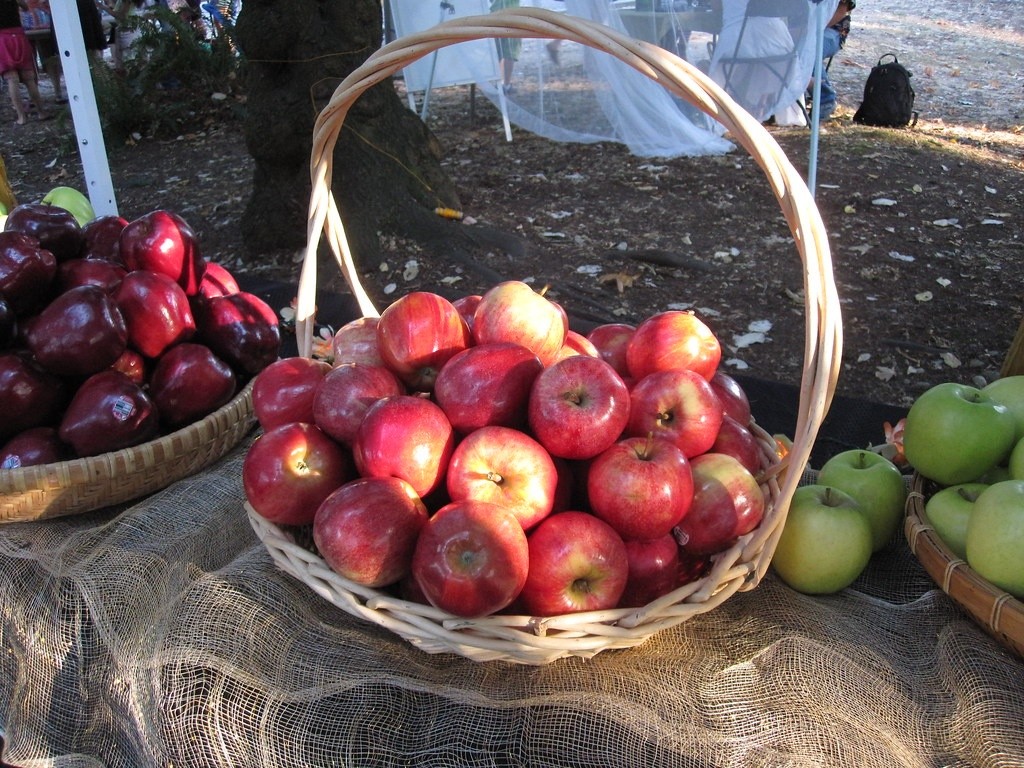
[903,325,1024,645]
[1,163,282,524]
[246,8,844,664]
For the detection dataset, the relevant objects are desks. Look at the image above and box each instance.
[616,9,723,126]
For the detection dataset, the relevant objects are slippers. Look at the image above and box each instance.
[29,99,36,108]
[54,96,68,104]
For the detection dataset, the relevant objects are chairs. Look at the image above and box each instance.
[706,0,834,133]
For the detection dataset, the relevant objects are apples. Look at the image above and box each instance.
[0,187,278,474]
[901,374,1024,609]
[239,281,765,620]
[771,484,873,595]
[817,448,905,558]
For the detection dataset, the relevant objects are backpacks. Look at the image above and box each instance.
[851,53,918,128]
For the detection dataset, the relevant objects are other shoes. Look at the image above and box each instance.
[808,98,837,121]
[504,84,518,95]
[547,42,561,66]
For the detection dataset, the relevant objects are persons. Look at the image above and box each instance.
[490,0,522,92]
[0,0,243,124]
[788,0,856,120]
[547,39,562,65]
